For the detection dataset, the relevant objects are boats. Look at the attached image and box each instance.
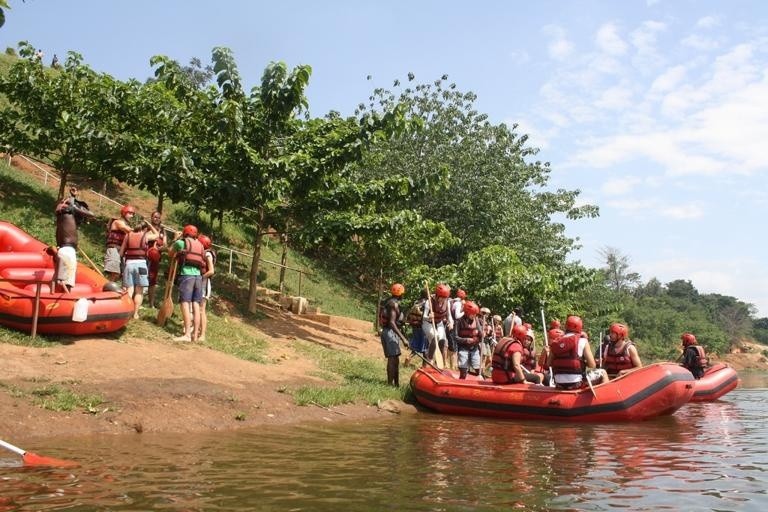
[409,362,695,423]
[673,400,747,511]
[412,410,698,506]
[0,219,134,337]
[484,362,738,403]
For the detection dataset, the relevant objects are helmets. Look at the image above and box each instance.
[610,323,627,336]
[512,323,532,337]
[436,283,466,300]
[147,247,161,263]
[682,334,695,345]
[552,320,560,327]
[183,224,211,249]
[464,300,502,322]
[391,284,404,296]
[121,205,136,217]
[566,315,583,333]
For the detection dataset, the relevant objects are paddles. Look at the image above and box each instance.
[1,440,40,465]
[540,306,555,387]
[158,255,178,328]
[425,281,444,370]
[585,361,596,398]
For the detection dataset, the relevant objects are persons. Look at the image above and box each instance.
[379,283,410,387]
[54,187,217,343]
[405,283,642,391]
[681,334,706,379]
